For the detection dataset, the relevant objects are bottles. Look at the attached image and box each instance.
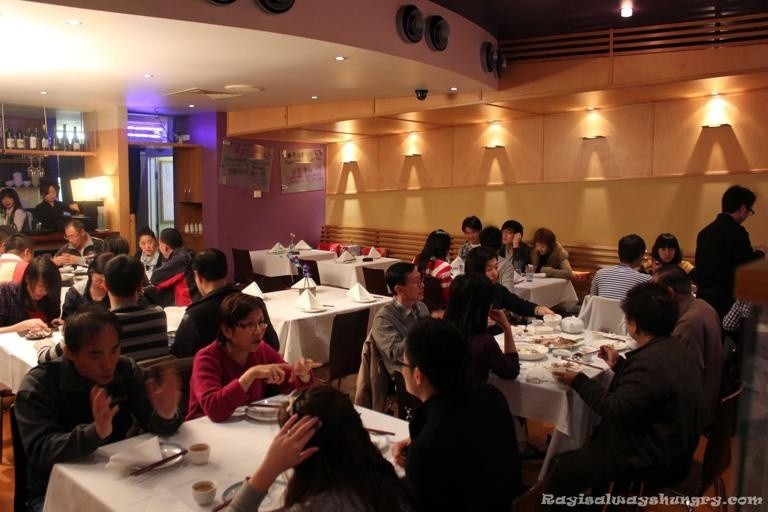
[51,127,60,150]
[16,128,26,149]
[61,124,69,150]
[39,124,49,150]
[5,126,15,149]
[71,127,80,151]
[29,128,37,149]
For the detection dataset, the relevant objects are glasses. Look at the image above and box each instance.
[409,278,424,286]
[395,356,410,369]
[63,231,80,239]
[236,320,268,332]
[747,206,755,216]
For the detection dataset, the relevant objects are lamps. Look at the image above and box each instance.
[128,121,170,140]
[449,87,460,92]
[616,1,640,18]
[334,56,348,61]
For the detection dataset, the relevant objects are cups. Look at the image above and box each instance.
[525,266,535,282]
[189,443,210,464]
[191,480,217,505]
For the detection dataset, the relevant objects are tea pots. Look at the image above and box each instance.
[562,315,585,332]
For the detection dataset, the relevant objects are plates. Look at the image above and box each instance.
[351,299,376,303]
[131,441,185,472]
[245,399,288,422]
[513,342,549,360]
[302,307,327,313]
[548,337,586,350]
[371,430,389,452]
[221,479,291,512]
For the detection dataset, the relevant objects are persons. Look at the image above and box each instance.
[412,229,452,319]
[170,248,279,398]
[133,227,167,282]
[51,221,104,267]
[371,261,430,391]
[531,228,572,280]
[103,235,150,287]
[500,220,531,273]
[654,264,723,419]
[459,216,482,260]
[695,184,766,437]
[104,255,169,363]
[444,274,520,383]
[0,225,18,257]
[0,233,36,284]
[392,315,523,511]
[151,229,202,306]
[0,257,61,333]
[591,234,654,299]
[15,306,184,512]
[640,233,697,294]
[35,181,80,232]
[226,385,417,512]
[51,253,115,329]
[185,294,323,423]
[533,281,706,512]
[0,188,30,235]
[481,227,518,296]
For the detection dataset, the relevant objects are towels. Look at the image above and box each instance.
[295,239,312,250]
[293,289,322,310]
[338,251,354,262]
[104,435,162,470]
[347,283,373,300]
[368,246,381,258]
[241,281,265,299]
[271,243,285,252]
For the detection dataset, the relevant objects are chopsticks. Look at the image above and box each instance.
[554,354,603,371]
[604,336,625,343]
[129,448,188,477]
[366,428,395,436]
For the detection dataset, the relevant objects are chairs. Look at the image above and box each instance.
[665,379,752,512]
[135,355,196,413]
[421,276,441,310]
[362,266,390,296]
[289,253,320,286]
[231,246,263,287]
[561,244,697,294]
[315,222,462,269]
[312,306,375,383]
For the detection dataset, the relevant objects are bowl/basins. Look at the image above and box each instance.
[532,319,543,326]
[535,326,554,335]
[543,313,562,327]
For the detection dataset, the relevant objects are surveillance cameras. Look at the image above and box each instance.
[413,89,429,101]
